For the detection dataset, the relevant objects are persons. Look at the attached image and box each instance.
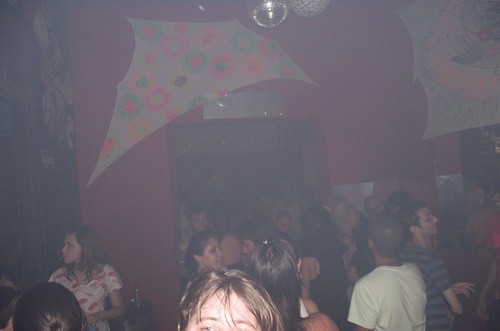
[0,176,500,331]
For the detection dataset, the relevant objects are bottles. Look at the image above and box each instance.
[125,289,153,331]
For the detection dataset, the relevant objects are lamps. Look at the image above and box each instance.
[247,0,289,29]
